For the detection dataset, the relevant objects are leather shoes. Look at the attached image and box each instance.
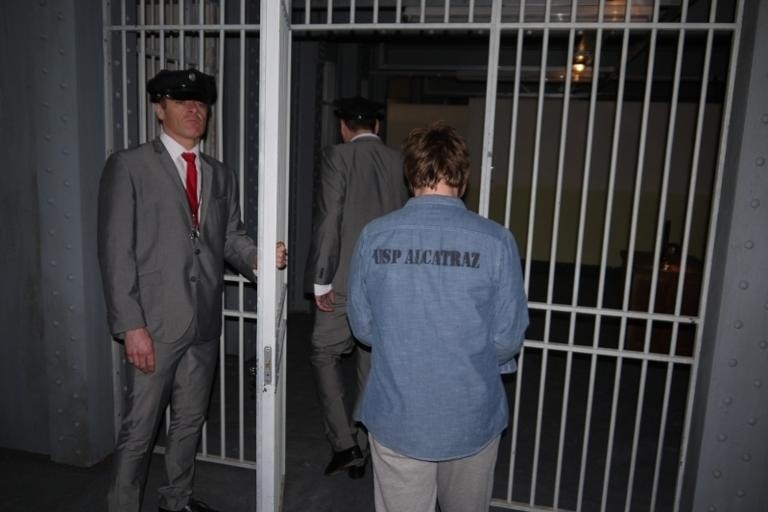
[322,445,362,478]
[347,465,365,480]
[157,497,220,512]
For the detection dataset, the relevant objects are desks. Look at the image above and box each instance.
[617,250,693,368]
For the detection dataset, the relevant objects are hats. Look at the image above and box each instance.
[146,68,219,105]
[333,96,384,121]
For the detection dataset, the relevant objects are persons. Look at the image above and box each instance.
[98,69,293,510]
[345,120,530,511]
[308,96,412,480]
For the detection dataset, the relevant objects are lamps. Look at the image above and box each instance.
[571,28,593,82]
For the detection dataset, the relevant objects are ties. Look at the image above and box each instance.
[180,152,202,237]
